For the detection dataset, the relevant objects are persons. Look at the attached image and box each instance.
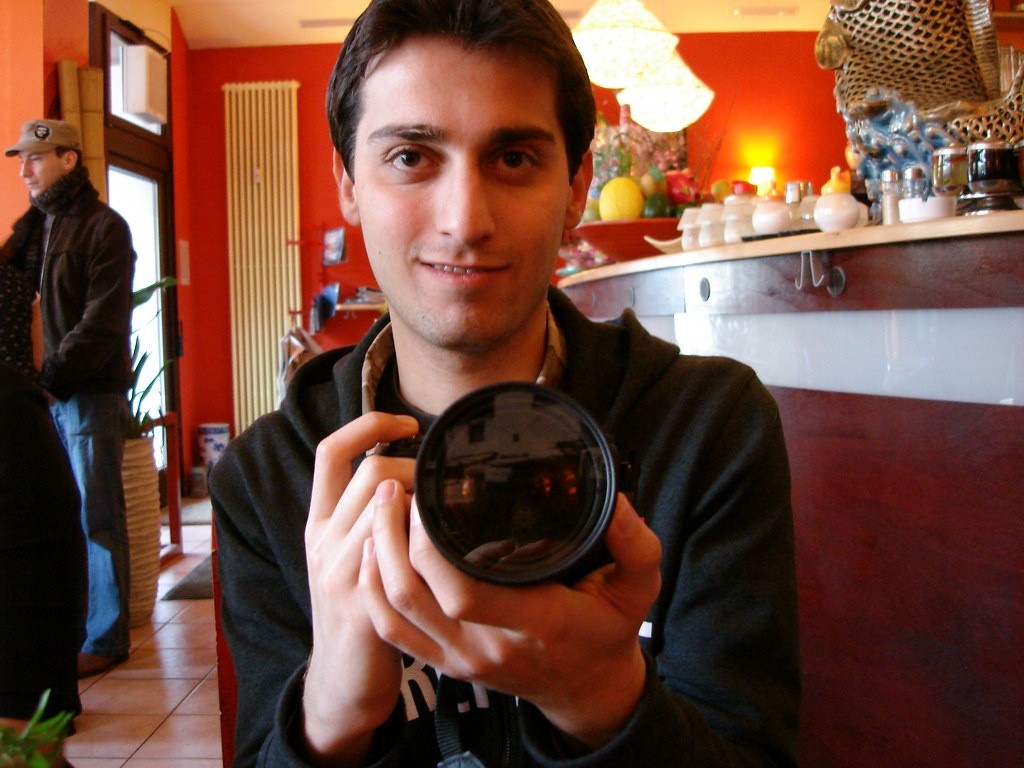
[209,0,800,768]
[0,119,139,675]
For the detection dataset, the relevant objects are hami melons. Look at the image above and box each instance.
[597,176,643,221]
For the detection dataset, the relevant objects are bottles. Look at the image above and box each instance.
[903,167,924,200]
[881,170,900,226]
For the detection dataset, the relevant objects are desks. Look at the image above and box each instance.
[335,304,388,310]
[140,412,183,566]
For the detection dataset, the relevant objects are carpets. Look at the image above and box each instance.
[160,553,214,601]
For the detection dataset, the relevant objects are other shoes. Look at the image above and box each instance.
[77,650,129,677]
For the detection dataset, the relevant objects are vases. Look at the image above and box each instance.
[197,424,230,471]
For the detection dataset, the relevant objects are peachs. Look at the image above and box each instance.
[640,171,667,196]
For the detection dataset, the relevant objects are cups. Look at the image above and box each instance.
[966,143,1019,213]
[931,148,968,216]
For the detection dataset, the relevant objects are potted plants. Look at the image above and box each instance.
[121,277,187,629]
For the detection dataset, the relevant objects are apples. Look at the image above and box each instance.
[581,199,601,222]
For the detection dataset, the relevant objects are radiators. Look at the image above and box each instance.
[222,78,302,437]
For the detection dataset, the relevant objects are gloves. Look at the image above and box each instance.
[36,363,73,403]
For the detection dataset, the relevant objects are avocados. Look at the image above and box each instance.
[642,191,692,218]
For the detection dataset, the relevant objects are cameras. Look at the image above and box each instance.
[367,380,634,592]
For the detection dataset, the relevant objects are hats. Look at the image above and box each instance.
[5,119,80,157]
